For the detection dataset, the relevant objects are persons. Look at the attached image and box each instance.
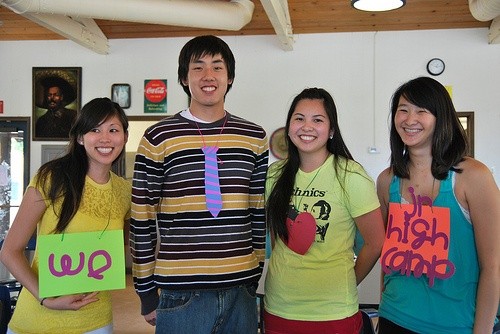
[0,98,131,334]
[376,77,500,334]
[130,34,269,334]
[263,87,385,334]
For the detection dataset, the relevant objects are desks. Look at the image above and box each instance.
[0,249,36,285]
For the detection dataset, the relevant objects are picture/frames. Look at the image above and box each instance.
[269,127,288,160]
[32,66,82,141]
[111,83,132,109]
[455,112,475,158]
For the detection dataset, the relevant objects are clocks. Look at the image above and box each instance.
[426,58,445,76]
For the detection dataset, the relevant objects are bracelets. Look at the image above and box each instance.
[41,298,47,305]
